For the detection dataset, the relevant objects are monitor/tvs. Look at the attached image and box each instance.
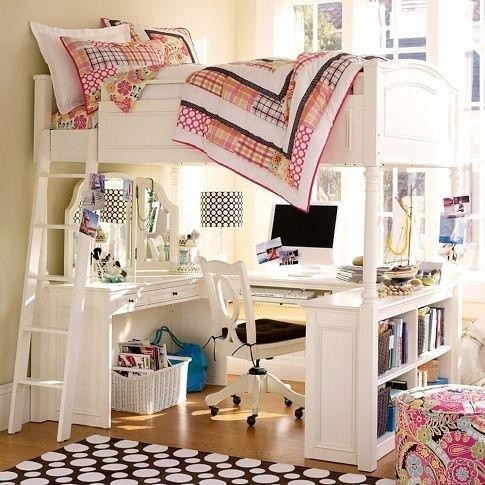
[267,201,340,277]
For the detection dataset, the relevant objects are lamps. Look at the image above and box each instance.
[199,189,245,260]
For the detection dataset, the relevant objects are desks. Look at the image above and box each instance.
[33,257,363,469]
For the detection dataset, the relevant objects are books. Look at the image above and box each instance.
[386,306,444,432]
[115,337,172,377]
[335,264,390,283]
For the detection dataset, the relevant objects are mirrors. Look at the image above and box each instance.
[62,171,180,279]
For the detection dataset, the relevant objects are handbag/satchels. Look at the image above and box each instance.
[148,326,210,394]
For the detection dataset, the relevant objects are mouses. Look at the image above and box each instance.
[323,292,332,296]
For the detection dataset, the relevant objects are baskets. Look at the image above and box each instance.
[111,354,192,416]
[377,328,390,376]
[418,313,431,357]
[420,358,441,384]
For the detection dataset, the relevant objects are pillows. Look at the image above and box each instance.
[26,17,199,116]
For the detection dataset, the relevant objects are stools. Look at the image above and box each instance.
[395,386,485,484]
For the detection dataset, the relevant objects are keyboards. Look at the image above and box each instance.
[238,288,315,300]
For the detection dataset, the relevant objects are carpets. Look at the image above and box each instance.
[0,433,395,485]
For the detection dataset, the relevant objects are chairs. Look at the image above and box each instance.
[196,254,305,425]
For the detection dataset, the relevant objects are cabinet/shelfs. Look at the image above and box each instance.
[300,276,462,476]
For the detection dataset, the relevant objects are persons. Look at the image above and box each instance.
[80,174,105,234]
[458,201,464,212]
[265,247,296,265]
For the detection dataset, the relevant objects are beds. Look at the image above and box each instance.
[34,65,462,170]
